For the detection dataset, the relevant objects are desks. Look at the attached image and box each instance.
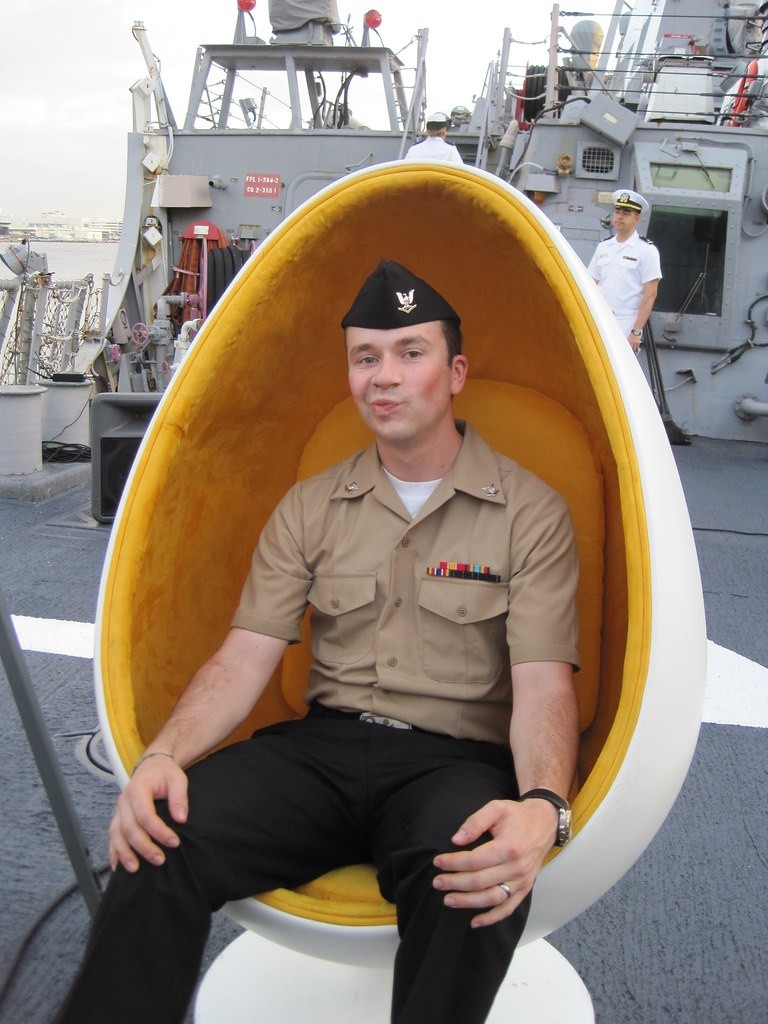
[180,43,410,137]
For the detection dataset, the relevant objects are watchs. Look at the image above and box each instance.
[518,789,571,847]
[631,329,641,336]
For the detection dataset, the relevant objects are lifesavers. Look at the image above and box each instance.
[725,61,759,127]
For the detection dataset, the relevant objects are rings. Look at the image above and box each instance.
[498,882,512,897]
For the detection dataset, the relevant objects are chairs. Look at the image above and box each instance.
[90,157,708,1024]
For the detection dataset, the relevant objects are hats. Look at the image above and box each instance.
[340,258,461,330]
[611,189,649,214]
[425,113,447,124]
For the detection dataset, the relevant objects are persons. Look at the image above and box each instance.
[587,189,663,355]
[405,114,465,164]
[50,256,586,1024]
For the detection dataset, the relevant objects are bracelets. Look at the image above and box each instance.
[128,752,175,779]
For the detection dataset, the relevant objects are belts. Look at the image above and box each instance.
[351,712,413,730]
